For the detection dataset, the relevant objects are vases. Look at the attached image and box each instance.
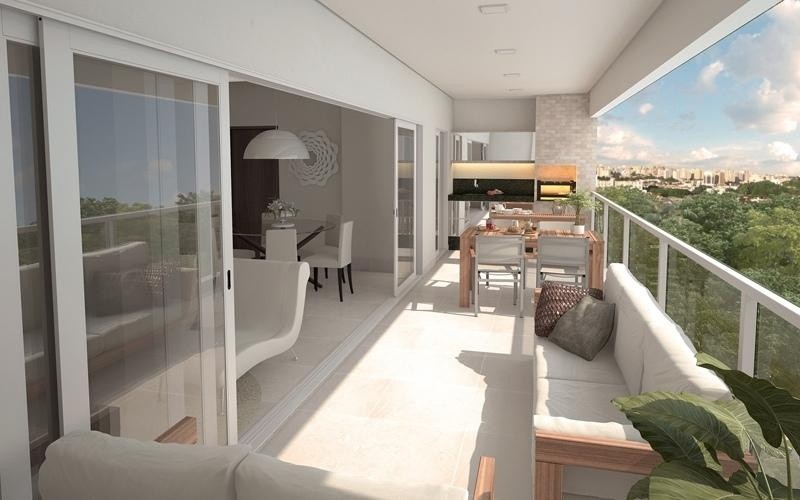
[553,199,566,215]
[272,210,297,219]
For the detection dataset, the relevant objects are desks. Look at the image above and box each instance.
[489,211,586,225]
[459,225,604,308]
[233,219,337,288]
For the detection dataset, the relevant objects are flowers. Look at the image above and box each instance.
[264,197,305,224]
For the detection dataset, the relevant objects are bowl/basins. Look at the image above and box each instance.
[503,207,531,215]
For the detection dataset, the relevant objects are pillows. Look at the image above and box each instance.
[90,268,154,317]
[548,293,616,362]
[535,279,603,336]
[133,258,177,307]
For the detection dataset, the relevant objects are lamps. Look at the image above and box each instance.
[242,93,310,161]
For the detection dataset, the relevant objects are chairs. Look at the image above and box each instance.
[265,229,297,262]
[186,258,310,416]
[470,233,526,318]
[536,233,590,288]
[302,213,354,302]
[165,220,256,280]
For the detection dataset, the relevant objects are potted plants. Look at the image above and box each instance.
[559,189,603,235]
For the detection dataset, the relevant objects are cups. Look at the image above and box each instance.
[512,220,518,228]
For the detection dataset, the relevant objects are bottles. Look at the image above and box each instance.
[486,218,493,230]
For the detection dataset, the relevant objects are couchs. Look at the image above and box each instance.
[36,415,495,500]
[531,261,759,500]
[20,242,199,402]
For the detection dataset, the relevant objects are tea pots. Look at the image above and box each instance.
[494,202,506,214]
